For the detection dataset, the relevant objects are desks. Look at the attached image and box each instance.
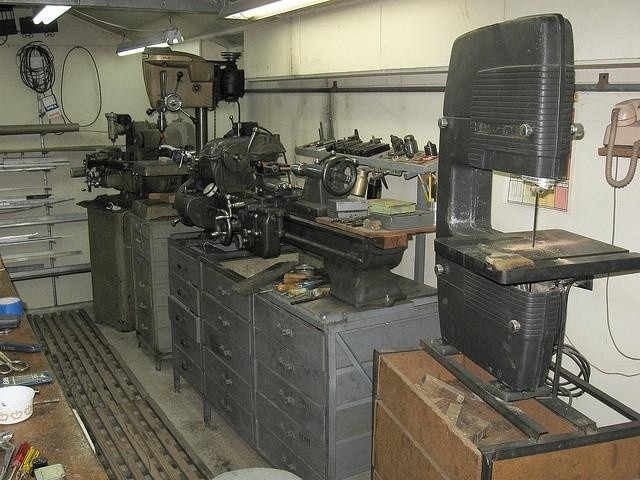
[0,253,112,480]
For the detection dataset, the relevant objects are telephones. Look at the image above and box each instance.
[603,99,640,147]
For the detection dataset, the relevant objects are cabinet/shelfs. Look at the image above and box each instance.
[252,266,440,476]
[166,226,249,419]
[200,252,286,456]
[125,204,208,373]
[366,338,640,480]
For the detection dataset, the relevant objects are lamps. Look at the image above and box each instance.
[113,15,185,57]
[29,3,73,26]
[214,0,339,21]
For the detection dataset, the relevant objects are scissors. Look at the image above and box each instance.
[0,352,29,374]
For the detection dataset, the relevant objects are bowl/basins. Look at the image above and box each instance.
[295,264,316,278]
[0,386,38,425]
[0,296,22,315]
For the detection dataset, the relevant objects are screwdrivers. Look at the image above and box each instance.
[10,442,39,480]
